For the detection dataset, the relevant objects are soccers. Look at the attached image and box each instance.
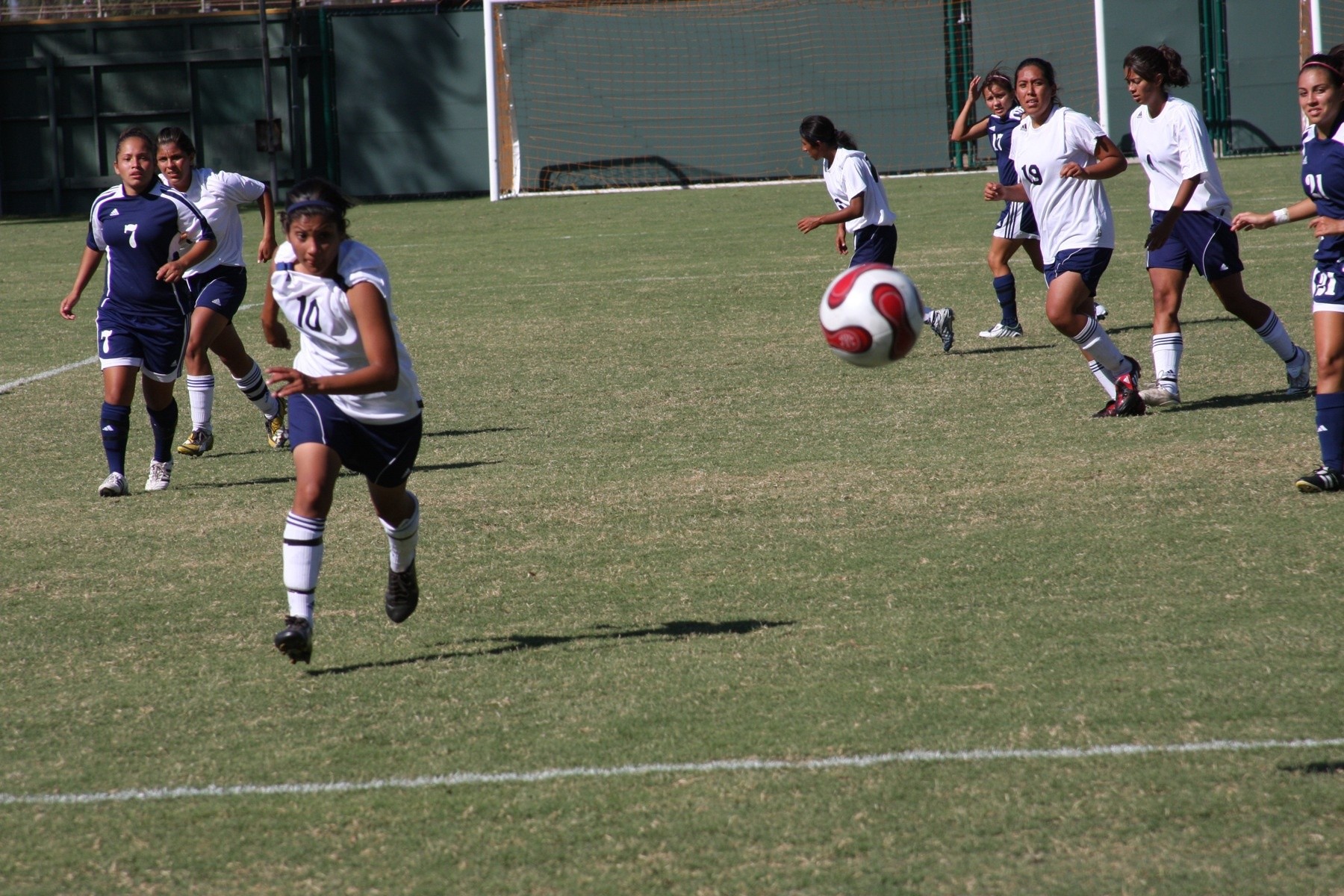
[817,263,926,370]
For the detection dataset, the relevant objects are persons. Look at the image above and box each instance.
[155,127,289,458]
[59,127,218,497]
[263,179,425,664]
[983,58,1146,419]
[1230,41,1344,496]
[950,61,1109,338]
[797,114,956,354]
[1122,45,1312,407]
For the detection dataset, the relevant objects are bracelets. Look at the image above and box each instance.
[1272,208,1291,225]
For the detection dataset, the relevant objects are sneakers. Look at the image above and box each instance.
[1137,370,1182,409]
[1287,346,1312,395]
[929,307,954,351]
[386,556,418,623]
[1115,355,1141,414]
[1296,462,1344,494]
[263,397,289,448]
[144,455,173,492]
[177,427,214,457]
[98,471,128,496]
[274,616,313,664]
[1092,399,1145,419]
[1094,302,1108,321]
[978,323,1023,338]
